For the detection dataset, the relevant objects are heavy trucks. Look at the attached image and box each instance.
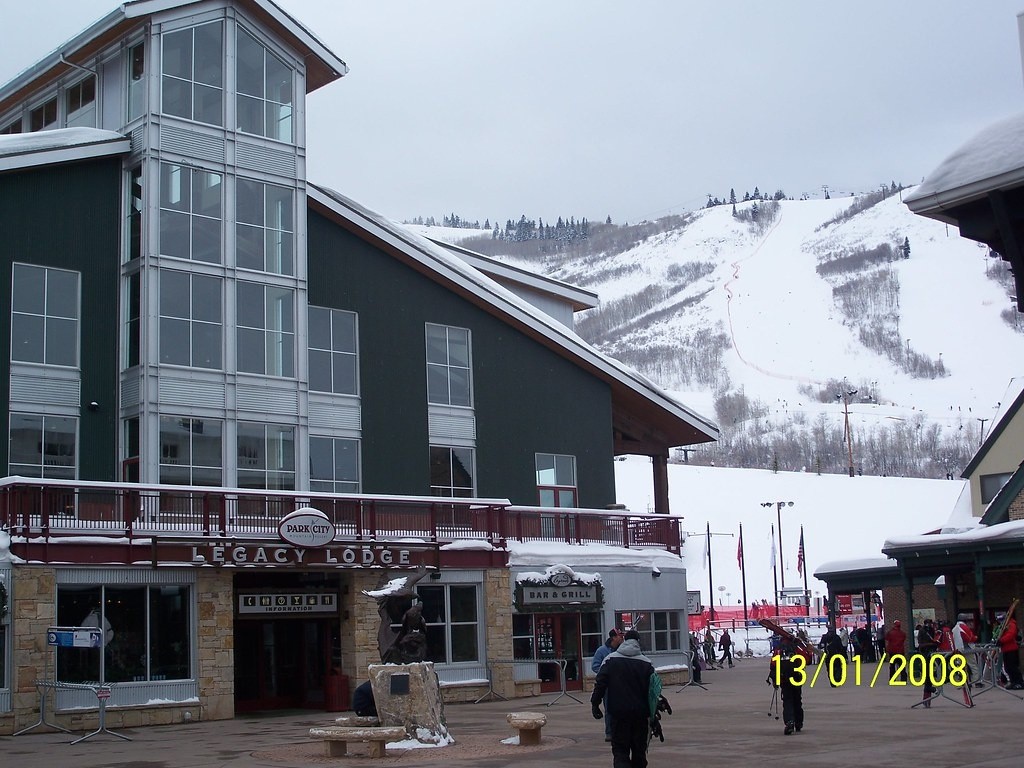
[747,589,884,627]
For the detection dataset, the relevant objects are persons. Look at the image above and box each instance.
[689,633,702,683]
[591,630,662,768]
[353,680,378,717]
[913,612,1024,690]
[823,595,828,615]
[770,634,804,735]
[885,620,908,684]
[704,630,716,663]
[591,626,626,742]
[749,602,759,618]
[717,628,735,668]
[770,625,886,664]
[818,627,848,687]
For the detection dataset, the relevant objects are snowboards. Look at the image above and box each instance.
[982,598,1020,679]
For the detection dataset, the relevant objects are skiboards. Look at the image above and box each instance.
[959,623,1006,688]
[942,627,973,705]
[758,618,823,656]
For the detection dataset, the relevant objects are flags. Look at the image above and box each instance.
[798,524,805,579]
[703,521,711,572]
[737,522,744,570]
[770,523,777,570]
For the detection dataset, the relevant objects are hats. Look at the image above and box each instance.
[995,613,1004,619]
[958,613,967,619]
[894,619,901,627]
[609,626,625,637]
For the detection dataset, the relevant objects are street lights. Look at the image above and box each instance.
[761,502,794,590]
[837,390,858,477]
[977,418,989,446]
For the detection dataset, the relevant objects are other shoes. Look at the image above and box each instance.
[1006,685,1023,690]
[784,727,792,735]
[729,664,735,668]
[795,721,803,732]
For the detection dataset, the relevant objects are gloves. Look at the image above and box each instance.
[592,704,603,720]
